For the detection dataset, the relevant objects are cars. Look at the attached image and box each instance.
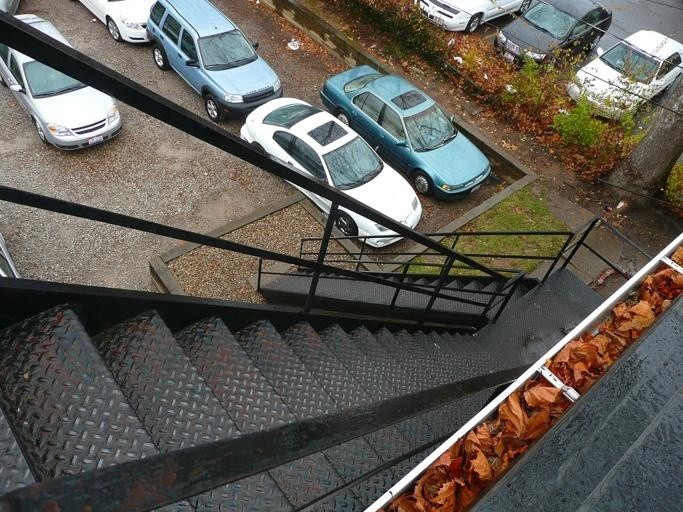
[564,30,682,122]
[493,1,612,72]
[319,64,492,202]
[412,0,532,35]
[239,95,423,249]
[0,13,124,151]
[76,1,157,46]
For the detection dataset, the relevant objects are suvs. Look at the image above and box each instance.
[146,1,283,124]
